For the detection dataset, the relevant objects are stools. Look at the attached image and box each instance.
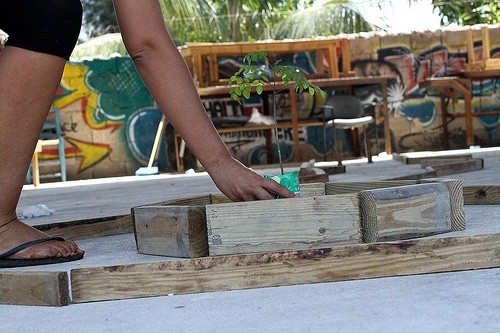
[466,26,500,72]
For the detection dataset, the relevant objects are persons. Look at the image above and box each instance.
[0,0,296,268]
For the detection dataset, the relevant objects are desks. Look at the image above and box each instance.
[419,73,500,149]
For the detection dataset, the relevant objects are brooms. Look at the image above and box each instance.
[135,113,168,177]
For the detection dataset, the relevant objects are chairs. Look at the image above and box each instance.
[323,93,379,161]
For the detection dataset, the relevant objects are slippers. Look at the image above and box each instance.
[1,235,83,268]
